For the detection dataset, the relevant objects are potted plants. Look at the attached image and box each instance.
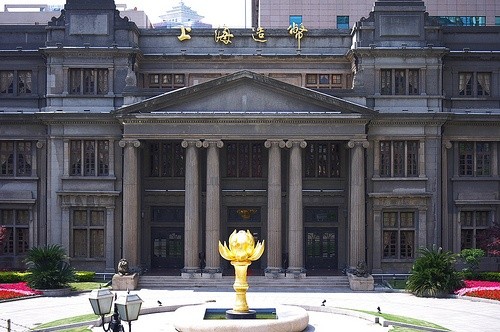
[21,242,78,297]
[406,244,466,298]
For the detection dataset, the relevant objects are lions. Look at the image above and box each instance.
[118,258,134,276]
[347,261,369,278]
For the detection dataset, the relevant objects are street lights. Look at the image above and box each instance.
[89,284,143,332]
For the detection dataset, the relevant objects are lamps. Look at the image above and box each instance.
[157,300,162,306]
[321,299,326,306]
[377,307,381,314]
